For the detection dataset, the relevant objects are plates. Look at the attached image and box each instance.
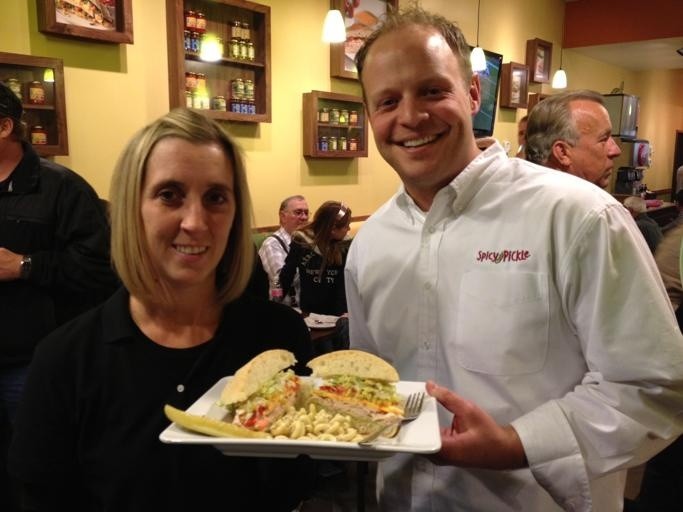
[157,374,443,462]
[303,315,339,328]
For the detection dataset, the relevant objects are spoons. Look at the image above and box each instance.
[314,319,335,324]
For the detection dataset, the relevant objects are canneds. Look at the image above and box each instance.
[6,77,24,103]
[231,78,256,115]
[213,95,228,112]
[184,10,208,52]
[228,21,256,62]
[185,72,209,110]
[320,107,359,151]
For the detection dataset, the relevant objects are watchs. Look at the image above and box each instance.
[20,252,32,278]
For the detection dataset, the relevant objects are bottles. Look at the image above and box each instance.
[330,108,338,122]
[184,8,256,64]
[340,109,349,124]
[348,111,357,124]
[319,137,327,151]
[185,71,255,115]
[328,136,337,151]
[320,107,328,122]
[347,139,359,151]
[5,78,22,100]
[338,137,346,151]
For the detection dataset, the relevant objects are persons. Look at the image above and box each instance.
[277,196,351,315]
[345,0,683,511]
[0,84,116,396]
[525,89,622,190]
[659,189,683,236]
[622,195,663,256]
[676,166,682,204]
[257,195,314,306]
[1,110,318,507]
[516,116,528,159]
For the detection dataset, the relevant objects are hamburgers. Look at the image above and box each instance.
[305,349,404,429]
[214,349,300,433]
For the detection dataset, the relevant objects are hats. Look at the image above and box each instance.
[0,84,24,118]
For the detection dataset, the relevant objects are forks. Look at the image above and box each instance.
[358,392,425,448]
